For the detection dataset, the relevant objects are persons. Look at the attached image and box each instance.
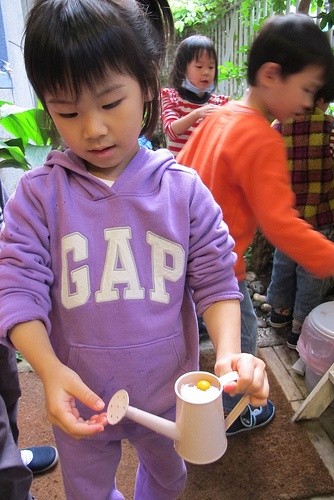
[0,185,58,500]
[0,0,270,500]
[176,14,334,435]
[266,49,334,348]
[160,35,229,158]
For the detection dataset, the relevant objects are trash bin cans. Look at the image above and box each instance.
[296,300,334,391]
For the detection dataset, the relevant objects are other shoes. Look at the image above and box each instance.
[20,445,58,473]
[287,329,301,349]
[269,307,293,327]
[226,399,276,435]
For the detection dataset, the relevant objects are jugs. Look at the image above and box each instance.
[107,372,250,465]
